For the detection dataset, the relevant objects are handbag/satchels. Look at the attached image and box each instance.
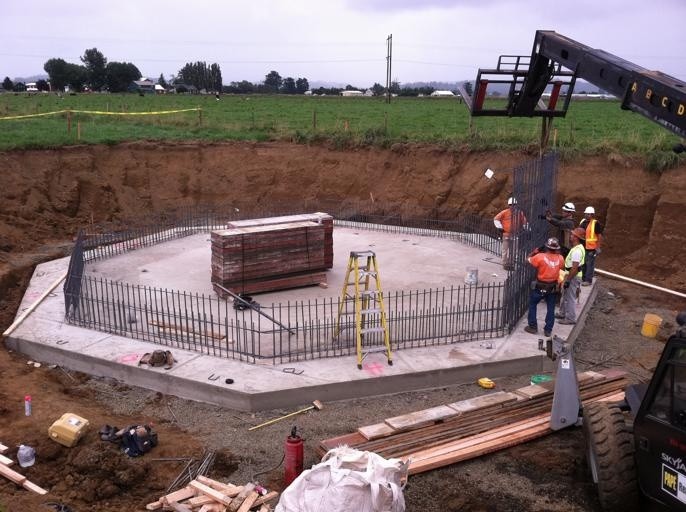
[534,283,554,297]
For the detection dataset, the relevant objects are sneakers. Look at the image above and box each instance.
[580,279,591,286]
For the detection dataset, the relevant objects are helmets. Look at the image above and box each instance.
[508,197,518,205]
[583,207,595,214]
[544,238,560,250]
[562,203,576,213]
[571,227,586,240]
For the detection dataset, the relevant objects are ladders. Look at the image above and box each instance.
[332,250,393,370]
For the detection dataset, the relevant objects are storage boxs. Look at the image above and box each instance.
[47,412,92,448]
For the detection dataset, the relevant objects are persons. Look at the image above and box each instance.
[579,206,602,286]
[494,197,531,271]
[524,237,565,337]
[555,227,586,324]
[546,202,576,261]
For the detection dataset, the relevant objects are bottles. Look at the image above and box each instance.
[16,444,36,468]
[24,395,32,416]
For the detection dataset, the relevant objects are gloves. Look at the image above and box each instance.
[563,281,570,290]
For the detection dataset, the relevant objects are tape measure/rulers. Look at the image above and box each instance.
[247,399,324,431]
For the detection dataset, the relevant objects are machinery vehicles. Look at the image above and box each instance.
[458,22,686,512]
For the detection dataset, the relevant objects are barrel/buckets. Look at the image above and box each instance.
[639,313,663,339]
[463,266,479,286]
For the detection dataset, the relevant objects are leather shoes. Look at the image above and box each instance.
[525,311,577,337]
